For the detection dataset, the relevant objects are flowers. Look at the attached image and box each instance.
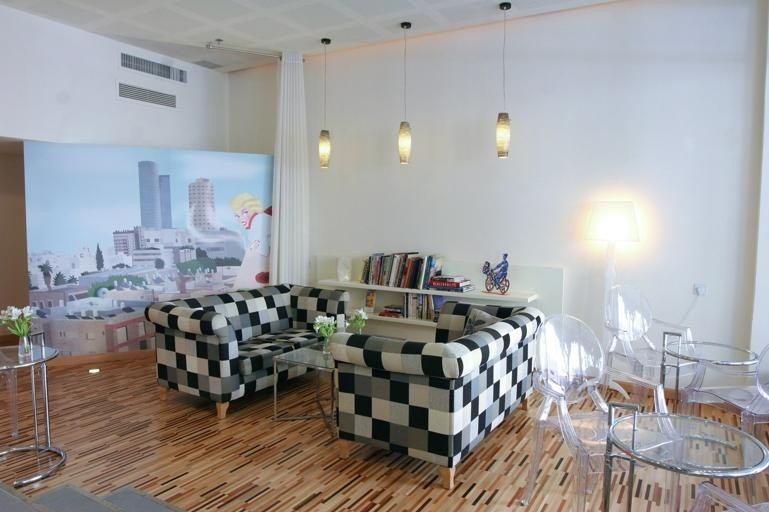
[0,306,34,353]
[350,308,368,331]
[314,314,335,342]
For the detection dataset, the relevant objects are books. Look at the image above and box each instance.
[430,273,465,283]
[383,292,444,320]
[360,251,427,293]
[423,253,445,289]
[429,280,471,288]
[428,283,475,293]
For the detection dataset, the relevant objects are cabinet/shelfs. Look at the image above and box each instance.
[318,278,539,328]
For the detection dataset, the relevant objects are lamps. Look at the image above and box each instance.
[496,2,512,158]
[398,20,411,165]
[318,38,331,169]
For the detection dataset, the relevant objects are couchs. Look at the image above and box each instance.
[325,302,546,491]
[145,283,351,421]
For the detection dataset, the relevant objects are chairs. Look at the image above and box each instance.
[1,312,45,438]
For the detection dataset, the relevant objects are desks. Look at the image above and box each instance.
[0,345,67,490]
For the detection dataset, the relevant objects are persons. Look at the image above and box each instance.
[491,253,509,291]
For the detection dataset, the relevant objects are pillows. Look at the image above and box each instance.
[463,309,502,338]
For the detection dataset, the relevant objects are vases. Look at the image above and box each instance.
[18,332,31,357]
[321,339,333,352]
[353,327,361,335]
